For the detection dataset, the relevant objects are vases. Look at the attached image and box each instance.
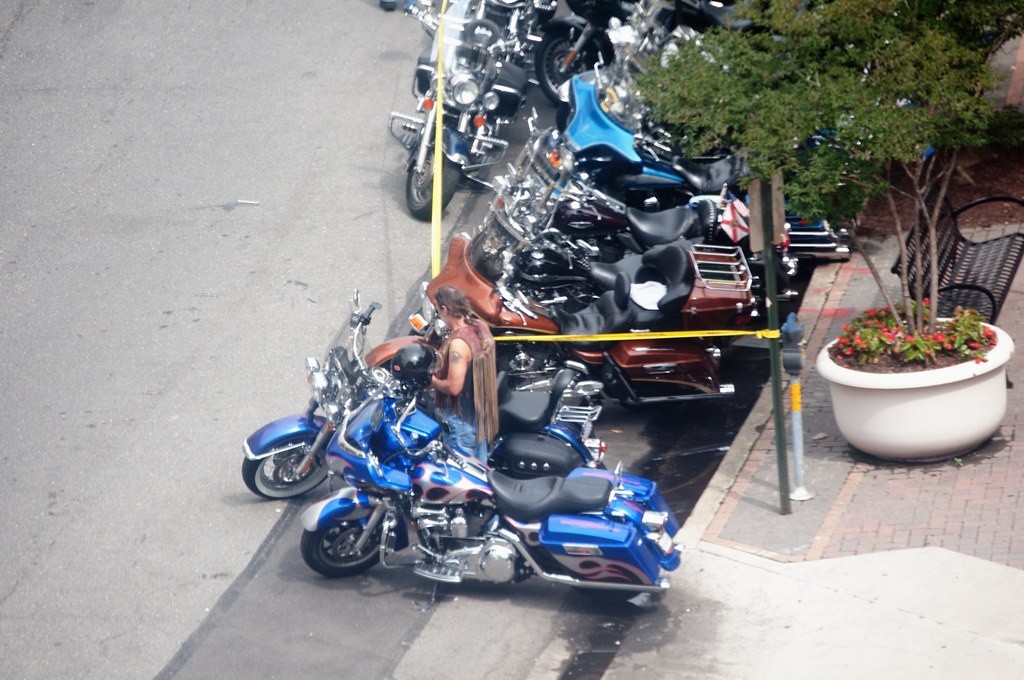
[816,316,1016,462]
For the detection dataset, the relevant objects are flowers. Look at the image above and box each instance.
[830,298,998,366]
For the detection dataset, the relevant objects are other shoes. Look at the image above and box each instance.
[404,8,412,14]
[380,1,397,10]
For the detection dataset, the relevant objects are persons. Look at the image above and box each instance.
[380,0,417,14]
[429,286,499,467]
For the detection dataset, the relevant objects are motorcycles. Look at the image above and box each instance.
[239,0,856,616]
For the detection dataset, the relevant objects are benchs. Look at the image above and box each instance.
[891,180,1024,388]
